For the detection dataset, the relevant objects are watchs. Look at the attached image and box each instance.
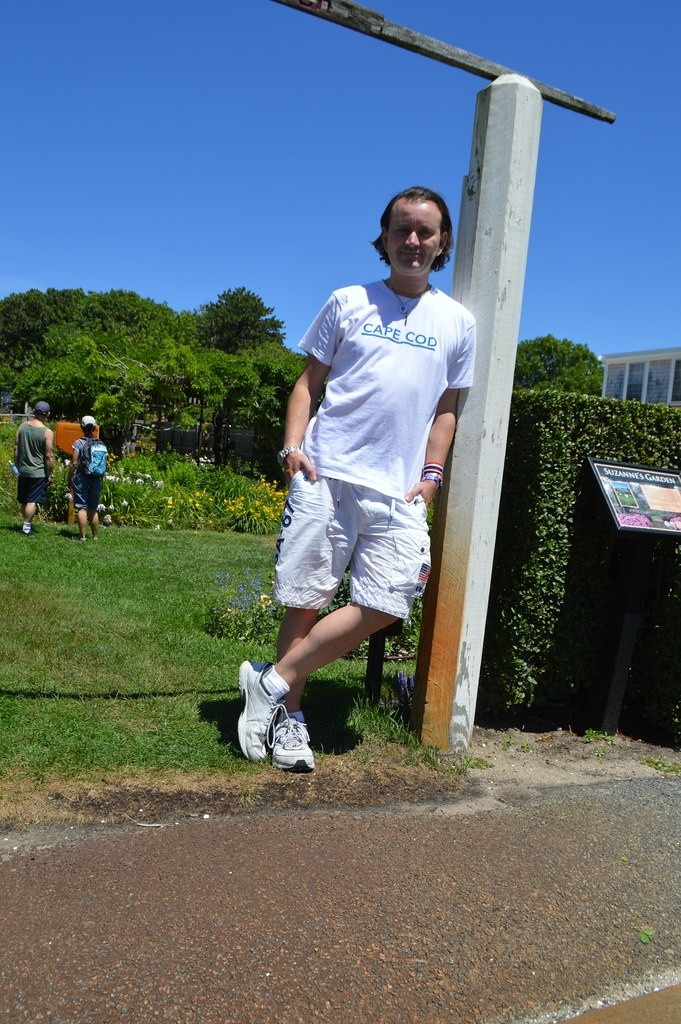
[277,447,301,467]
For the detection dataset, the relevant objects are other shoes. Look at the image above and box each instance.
[21,526,39,536]
[72,535,87,542]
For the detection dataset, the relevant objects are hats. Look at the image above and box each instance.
[35,401,50,414]
[82,415,97,427]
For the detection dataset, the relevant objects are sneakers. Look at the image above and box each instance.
[272,718,315,774]
[238,661,291,762]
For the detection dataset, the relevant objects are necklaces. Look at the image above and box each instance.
[384,277,430,314]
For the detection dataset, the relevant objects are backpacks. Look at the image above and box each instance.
[77,438,107,477]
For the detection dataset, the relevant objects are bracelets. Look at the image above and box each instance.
[421,462,444,490]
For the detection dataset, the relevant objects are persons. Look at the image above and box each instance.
[238,185,476,774]
[67,415,108,542]
[14,401,54,537]
